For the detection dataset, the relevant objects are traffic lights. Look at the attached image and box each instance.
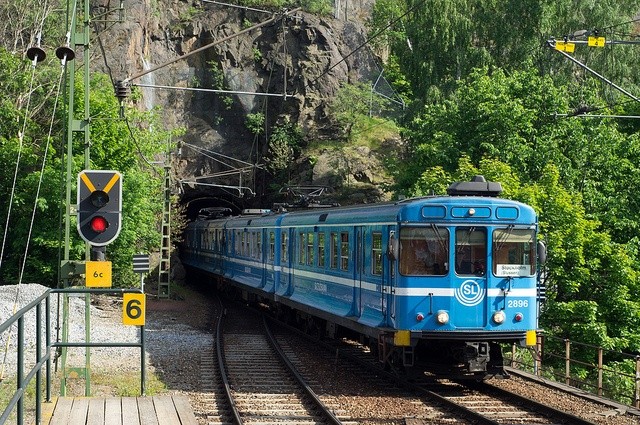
[76,170,122,247]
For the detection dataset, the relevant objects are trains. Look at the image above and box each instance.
[172,174,547,380]
